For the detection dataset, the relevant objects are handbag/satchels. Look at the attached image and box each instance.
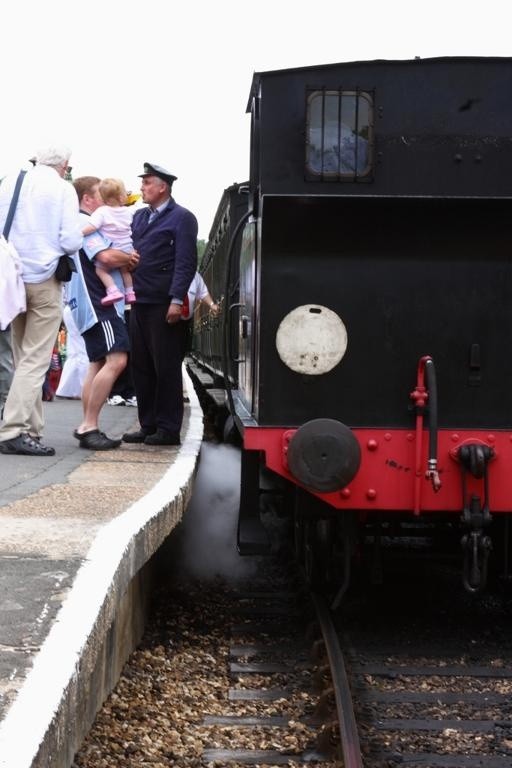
[57,256,76,282]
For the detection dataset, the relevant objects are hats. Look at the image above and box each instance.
[138,162,177,184]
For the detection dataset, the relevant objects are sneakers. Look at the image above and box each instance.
[101,292,123,305]
[108,395,125,406]
[144,430,180,445]
[126,396,137,407]
[80,433,120,450]
[1,433,54,456]
[126,292,136,304]
[122,430,145,442]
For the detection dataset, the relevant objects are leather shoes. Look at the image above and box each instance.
[184,396,189,402]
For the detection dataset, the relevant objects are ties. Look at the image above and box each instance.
[182,294,189,317]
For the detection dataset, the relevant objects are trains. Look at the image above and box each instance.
[184,52,512,619]
